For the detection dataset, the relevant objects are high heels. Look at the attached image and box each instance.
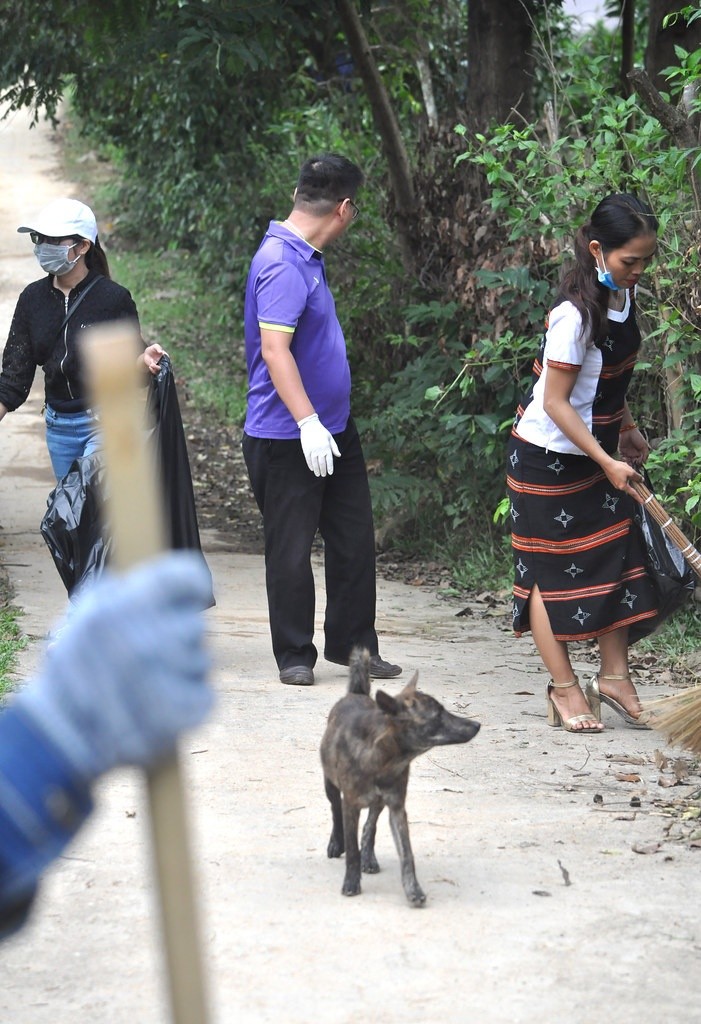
[585,672,657,729]
[546,675,601,733]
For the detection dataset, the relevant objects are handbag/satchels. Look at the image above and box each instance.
[628,462,696,646]
[41,354,216,620]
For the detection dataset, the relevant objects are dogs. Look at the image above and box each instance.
[317,644,481,908]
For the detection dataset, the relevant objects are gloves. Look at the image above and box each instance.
[297,413,341,478]
[21,548,216,780]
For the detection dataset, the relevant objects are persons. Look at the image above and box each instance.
[0,199,168,484]
[0,551,215,937]
[242,153,402,683]
[506,195,658,733]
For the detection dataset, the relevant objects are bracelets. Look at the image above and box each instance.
[620,423,637,431]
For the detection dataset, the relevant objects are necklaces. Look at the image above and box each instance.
[615,293,620,309]
[284,220,305,239]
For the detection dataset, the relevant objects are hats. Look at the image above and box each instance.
[16,199,98,245]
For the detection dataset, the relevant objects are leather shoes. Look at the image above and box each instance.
[280,665,314,684]
[324,654,402,676]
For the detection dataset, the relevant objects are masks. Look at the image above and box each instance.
[594,250,622,290]
[33,243,81,277]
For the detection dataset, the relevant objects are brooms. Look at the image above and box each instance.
[628,681,701,765]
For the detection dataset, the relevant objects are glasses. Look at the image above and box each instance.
[30,233,77,245]
[337,198,359,219]
